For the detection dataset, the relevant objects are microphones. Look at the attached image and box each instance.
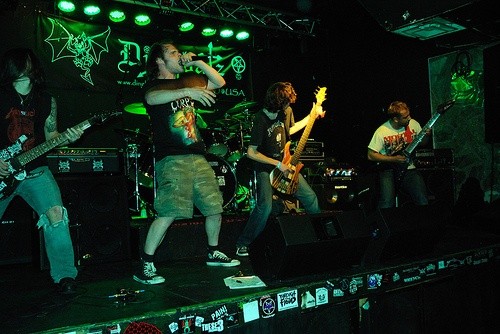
[179,56,211,65]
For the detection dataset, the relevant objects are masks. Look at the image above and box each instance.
[13,77,33,95]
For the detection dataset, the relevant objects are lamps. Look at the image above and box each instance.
[52,0,258,48]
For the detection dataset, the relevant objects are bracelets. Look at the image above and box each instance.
[276,161,282,169]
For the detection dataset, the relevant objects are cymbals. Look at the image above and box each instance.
[216,119,237,126]
[227,102,257,113]
[124,102,149,115]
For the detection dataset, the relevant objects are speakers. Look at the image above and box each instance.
[250,206,453,282]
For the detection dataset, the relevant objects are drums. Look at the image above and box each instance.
[225,138,242,163]
[202,132,228,157]
[201,153,237,208]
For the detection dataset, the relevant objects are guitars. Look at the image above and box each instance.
[384,100,454,188]
[0,108,122,202]
[268,85,328,198]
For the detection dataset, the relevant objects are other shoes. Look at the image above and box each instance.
[59,278,77,294]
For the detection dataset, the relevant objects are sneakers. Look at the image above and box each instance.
[206,251,241,266]
[231,237,249,256]
[132,257,165,285]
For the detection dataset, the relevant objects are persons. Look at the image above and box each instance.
[132,40,239,285]
[0,43,85,295]
[234,81,326,258]
[366,100,430,208]
